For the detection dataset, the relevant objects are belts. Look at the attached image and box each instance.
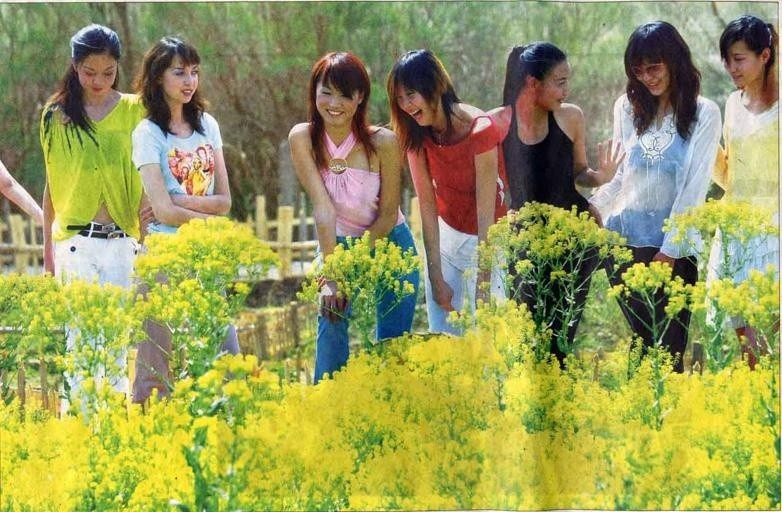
[66,222,130,239]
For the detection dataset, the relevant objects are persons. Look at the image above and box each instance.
[487,40,626,369]
[40,24,146,420]
[288,52,420,385]
[711,14,778,369]
[129,35,247,404]
[388,49,507,337]
[0,159,44,227]
[588,20,722,373]
